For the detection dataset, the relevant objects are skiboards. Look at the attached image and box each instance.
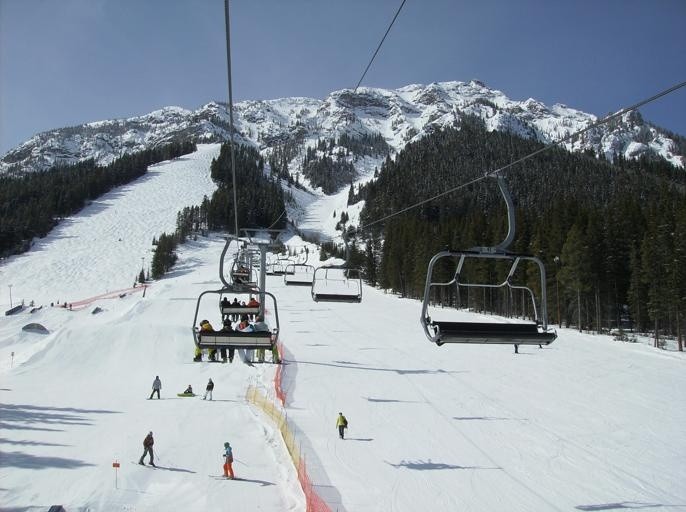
[131,461,160,470]
[207,476,245,480]
[185,358,287,367]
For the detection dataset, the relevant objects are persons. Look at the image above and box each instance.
[336,412,345,439]
[139,432,155,466]
[194,297,282,366]
[184,385,192,394]
[148,376,161,400]
[223,442,234,480]
[201,378,214,401]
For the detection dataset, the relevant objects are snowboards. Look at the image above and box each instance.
[178,393,195,396]
[146,397,163,400]
